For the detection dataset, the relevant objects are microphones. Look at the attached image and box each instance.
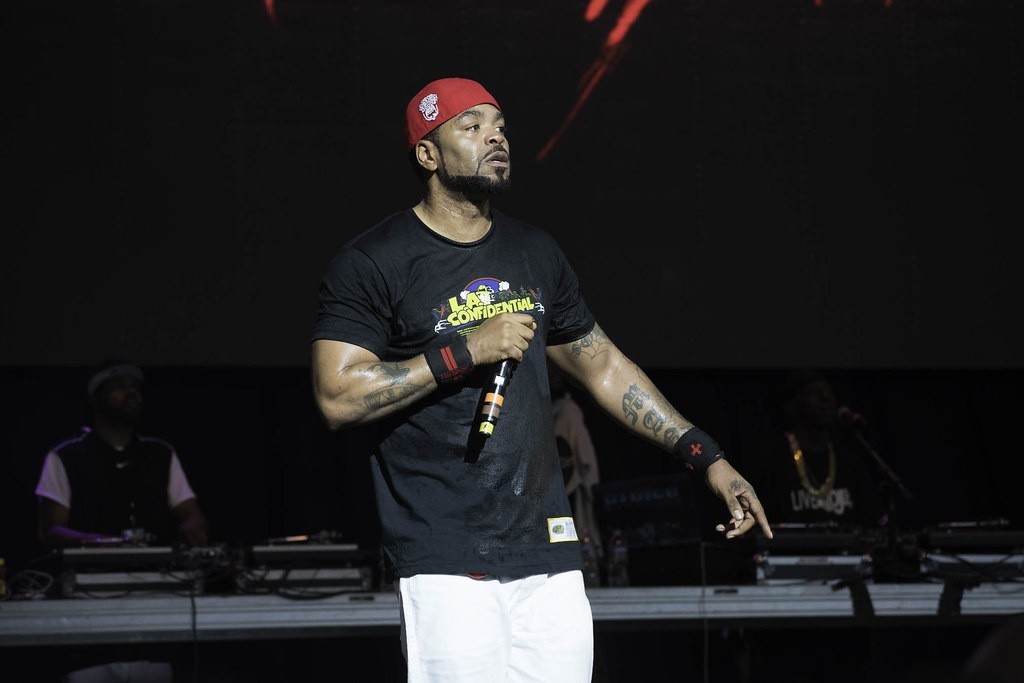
[837,408,868,428]
[479,359,518,437]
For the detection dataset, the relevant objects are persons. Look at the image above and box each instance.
[34,368,202,683]
[310,78,773,683]
[745,366,891,683]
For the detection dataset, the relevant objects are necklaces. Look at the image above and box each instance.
[786,431,838,498]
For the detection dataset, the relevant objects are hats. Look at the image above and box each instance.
[403,77,504,156]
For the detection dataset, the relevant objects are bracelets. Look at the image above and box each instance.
[671,427,724,476]
[422,333,474,389]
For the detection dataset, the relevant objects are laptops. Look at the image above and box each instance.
[592,472,701,548]
